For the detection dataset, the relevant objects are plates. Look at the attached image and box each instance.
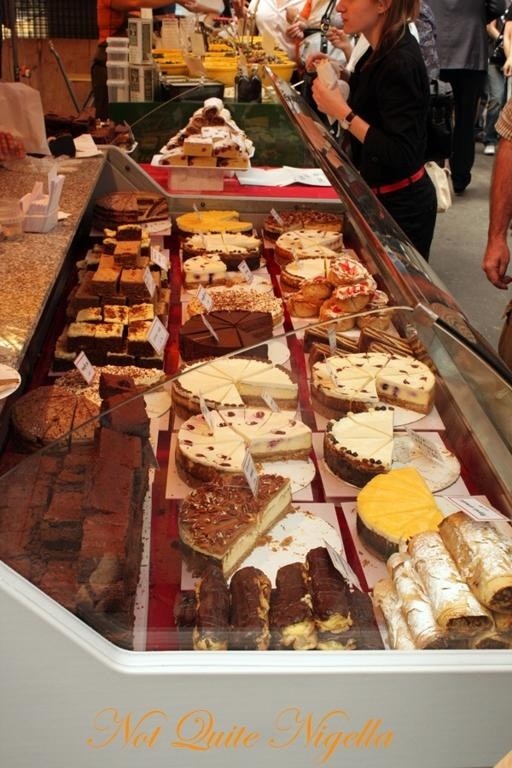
[0,362,22,404]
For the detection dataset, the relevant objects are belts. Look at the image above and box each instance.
[367,164,428,199]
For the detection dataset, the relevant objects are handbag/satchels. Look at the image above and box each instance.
[246,13,258,36]
[297,28,361,72]
[421,158,456,215]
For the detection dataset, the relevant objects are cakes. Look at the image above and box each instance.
[158,124,250,168]
[1,191,446,652]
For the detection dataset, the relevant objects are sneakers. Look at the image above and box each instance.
[481,142,496,156]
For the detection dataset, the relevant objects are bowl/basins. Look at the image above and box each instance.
[151,34,298,87]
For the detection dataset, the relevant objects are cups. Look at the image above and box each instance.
[1,200,26,242]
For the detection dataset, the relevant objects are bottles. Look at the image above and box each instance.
[234,62,263,104]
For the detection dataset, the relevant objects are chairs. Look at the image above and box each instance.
[25,176,68,236]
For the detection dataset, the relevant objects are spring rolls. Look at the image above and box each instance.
[373,510,512,650]
[166,96,254,155]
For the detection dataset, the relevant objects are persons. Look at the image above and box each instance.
[483,98,511,372]
